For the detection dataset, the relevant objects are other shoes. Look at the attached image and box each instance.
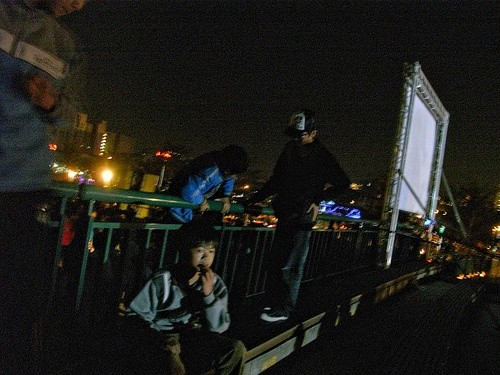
[260,307,288,321]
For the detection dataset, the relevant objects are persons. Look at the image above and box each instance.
[118,145,249,316]
[0,0,89,375]
[240,110,351,322]
[122,219,246,375]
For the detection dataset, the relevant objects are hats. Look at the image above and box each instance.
[283,110,315,137]
